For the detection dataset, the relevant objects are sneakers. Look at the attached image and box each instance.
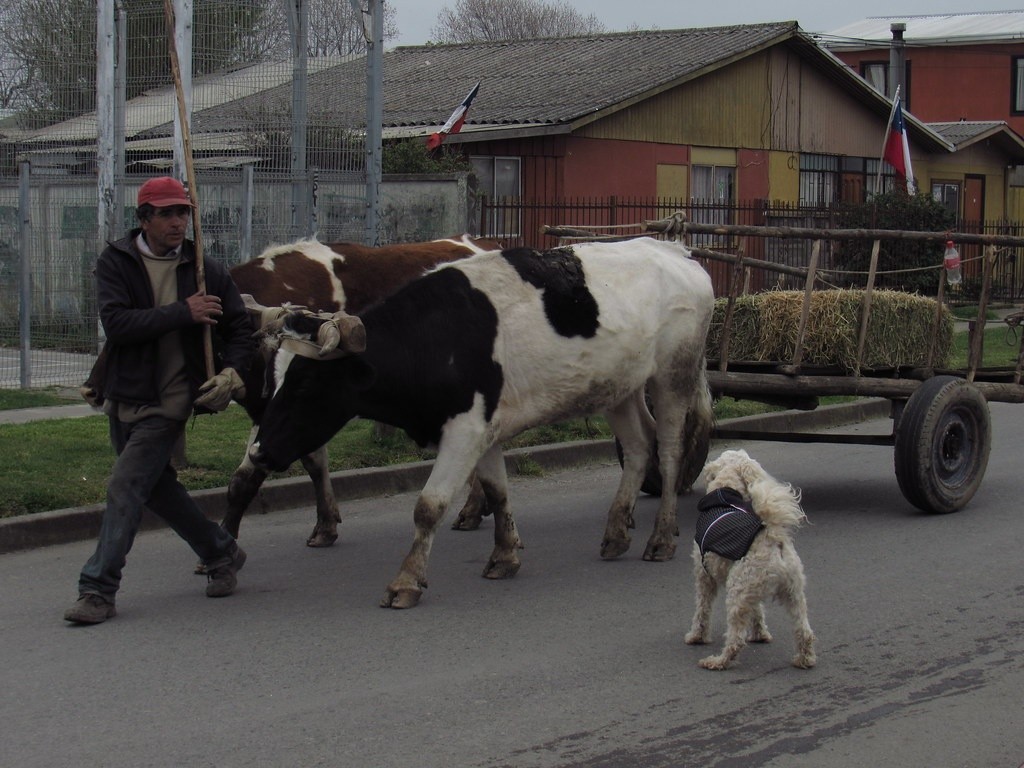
[63,594,117,621]
[204,544,246,596]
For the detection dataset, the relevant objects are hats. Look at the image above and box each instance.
[138,176,197,209]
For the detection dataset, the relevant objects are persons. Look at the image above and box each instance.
[64,177,251,624]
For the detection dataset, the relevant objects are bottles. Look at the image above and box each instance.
[945,240,962,286]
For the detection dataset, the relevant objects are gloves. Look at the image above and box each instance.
[192,368,243,409]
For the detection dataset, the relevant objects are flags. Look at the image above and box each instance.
[884,88,916,196]
[424,80,480,151]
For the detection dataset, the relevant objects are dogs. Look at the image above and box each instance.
[683,448,820,670]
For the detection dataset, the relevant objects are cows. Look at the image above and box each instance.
[194,235,716,611]
